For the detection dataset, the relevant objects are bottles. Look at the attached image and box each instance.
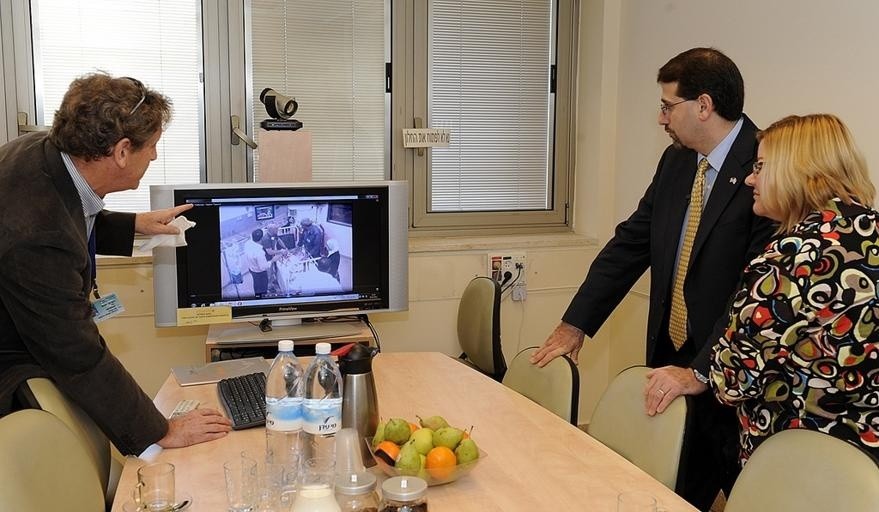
[304,343,343,499]
[265,339,305,492]
[334,469,429,512]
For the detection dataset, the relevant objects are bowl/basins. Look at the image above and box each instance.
[365,424,489,488]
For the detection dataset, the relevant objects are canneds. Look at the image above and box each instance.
[334,473,380,512]
[377,475,431,512]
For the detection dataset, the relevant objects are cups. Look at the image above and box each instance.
[223,442,336,512]
[334,427,365,472]
[131,460,176,510]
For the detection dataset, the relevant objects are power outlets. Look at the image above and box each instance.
[487,250,528,286]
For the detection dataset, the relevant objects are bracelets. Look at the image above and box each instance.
[692,367,709,385]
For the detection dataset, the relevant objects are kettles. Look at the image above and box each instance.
[335,343,380,468]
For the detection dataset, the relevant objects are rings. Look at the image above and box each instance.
[657,388,666,395]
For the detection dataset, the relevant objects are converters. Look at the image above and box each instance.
[512,283,527,301]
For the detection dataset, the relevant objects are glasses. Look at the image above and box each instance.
[661,99,687,115]
[753,163,767,176]
[117,77,147,115]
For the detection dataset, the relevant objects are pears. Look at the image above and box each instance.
[371,416,478,482]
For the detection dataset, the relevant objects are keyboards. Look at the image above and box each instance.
[217,372,268,431]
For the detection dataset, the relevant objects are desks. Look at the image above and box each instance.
[108,351,699,512]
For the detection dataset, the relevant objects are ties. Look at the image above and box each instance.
[668,159,710,352]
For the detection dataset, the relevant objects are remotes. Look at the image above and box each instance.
[169,399,200,420]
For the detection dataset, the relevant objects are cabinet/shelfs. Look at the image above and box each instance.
[202,319,373,363]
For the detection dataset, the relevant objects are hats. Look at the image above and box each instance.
[301,218,312,225]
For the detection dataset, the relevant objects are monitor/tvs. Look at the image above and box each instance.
[149,180,411,328]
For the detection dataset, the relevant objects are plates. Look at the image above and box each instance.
[122,491,192,512]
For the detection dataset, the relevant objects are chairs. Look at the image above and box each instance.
[0,380,121,512]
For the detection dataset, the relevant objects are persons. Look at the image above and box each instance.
[2,72,234,458]
[242,212,343,294]
[530,47,766,512]
[708,111,879,470]
